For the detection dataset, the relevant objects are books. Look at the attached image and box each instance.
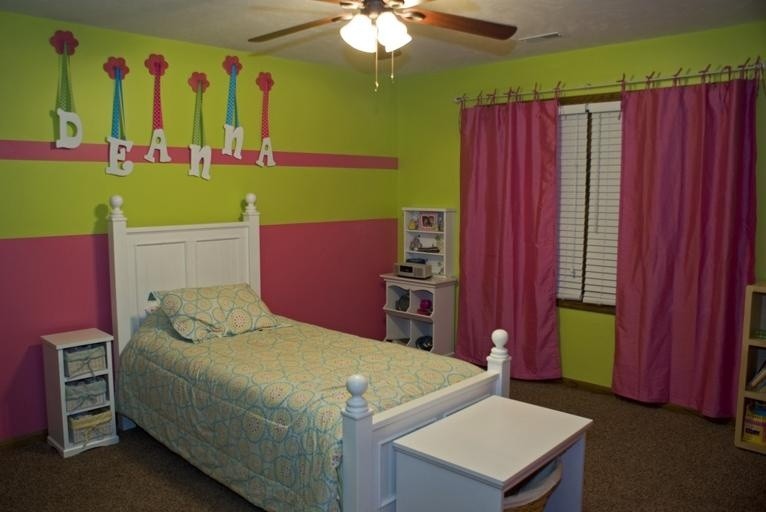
[746,365,766,393]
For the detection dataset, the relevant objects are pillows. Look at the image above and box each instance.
[146,275,294,344]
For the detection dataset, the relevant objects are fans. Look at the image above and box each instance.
[248,0,518,43]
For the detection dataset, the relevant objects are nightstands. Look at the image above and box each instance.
[40,327,120,460]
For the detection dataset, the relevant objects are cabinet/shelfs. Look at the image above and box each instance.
[392,396,594,510]
[733,283,766,455]
[378,206,457,358]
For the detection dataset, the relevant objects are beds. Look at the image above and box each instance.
[103,192,514,511]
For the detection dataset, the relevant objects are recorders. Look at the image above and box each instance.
[394,262,433,278]
